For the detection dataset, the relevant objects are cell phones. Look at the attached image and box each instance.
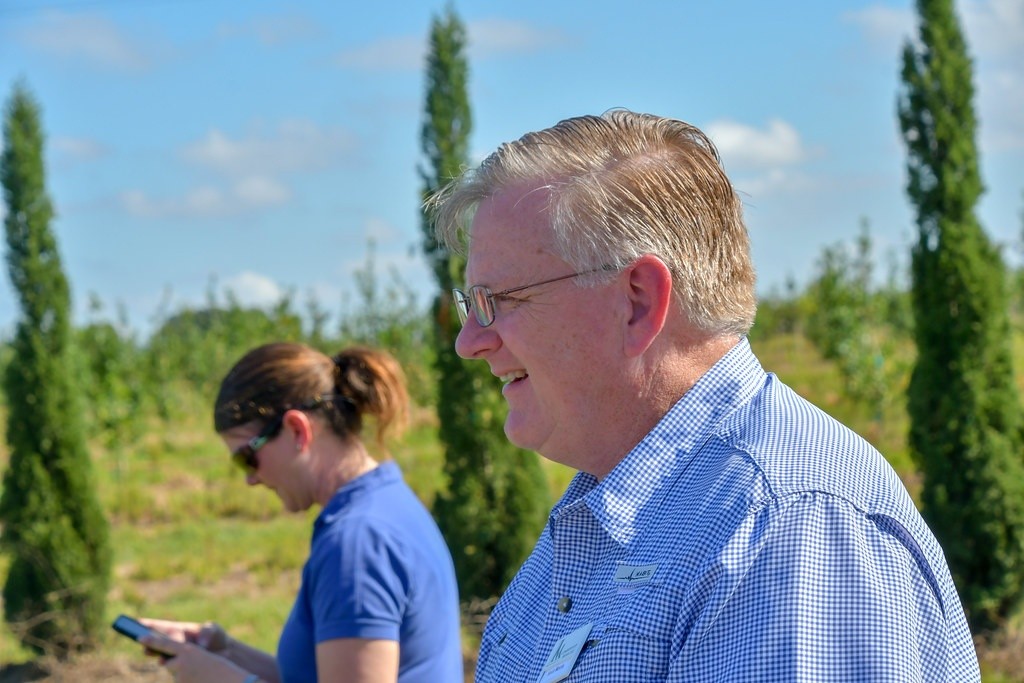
[111,613,175,660]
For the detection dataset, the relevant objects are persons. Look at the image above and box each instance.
[135,340,463,683]
[420,112,983,683]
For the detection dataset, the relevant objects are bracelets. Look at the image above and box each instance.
[245,675,257,683]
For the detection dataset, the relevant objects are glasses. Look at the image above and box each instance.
[452,257,631,328]
[233,401,323,473]
